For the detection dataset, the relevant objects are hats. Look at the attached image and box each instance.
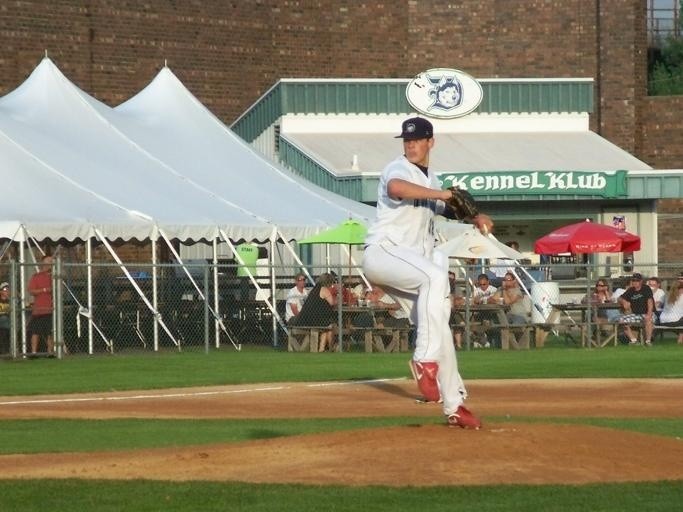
[630,273,644,282]
[394,117,433,140]
[0,281,9,290]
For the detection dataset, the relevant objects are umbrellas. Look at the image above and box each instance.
[295,219,369,308]
[433,226,527,351]
[534,217,640,349]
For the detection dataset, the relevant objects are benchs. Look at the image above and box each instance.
[284,277,683,352]
[61,277,314,354]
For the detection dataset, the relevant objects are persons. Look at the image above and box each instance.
[27,253,57,359]
[361,118,494,428]
[0,281,32,313]
[580,272,683,348]
[284,269,528,353]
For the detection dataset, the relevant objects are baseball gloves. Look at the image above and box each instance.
[447,186,479,220]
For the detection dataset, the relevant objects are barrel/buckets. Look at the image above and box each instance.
[531,281,560,324]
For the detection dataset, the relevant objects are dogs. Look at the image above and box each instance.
[435,74,461,109]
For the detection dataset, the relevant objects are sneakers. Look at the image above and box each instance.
[446,406,482,430]
[453,340,491,350]
[407,359,440,402]
[629,337,653,347]
